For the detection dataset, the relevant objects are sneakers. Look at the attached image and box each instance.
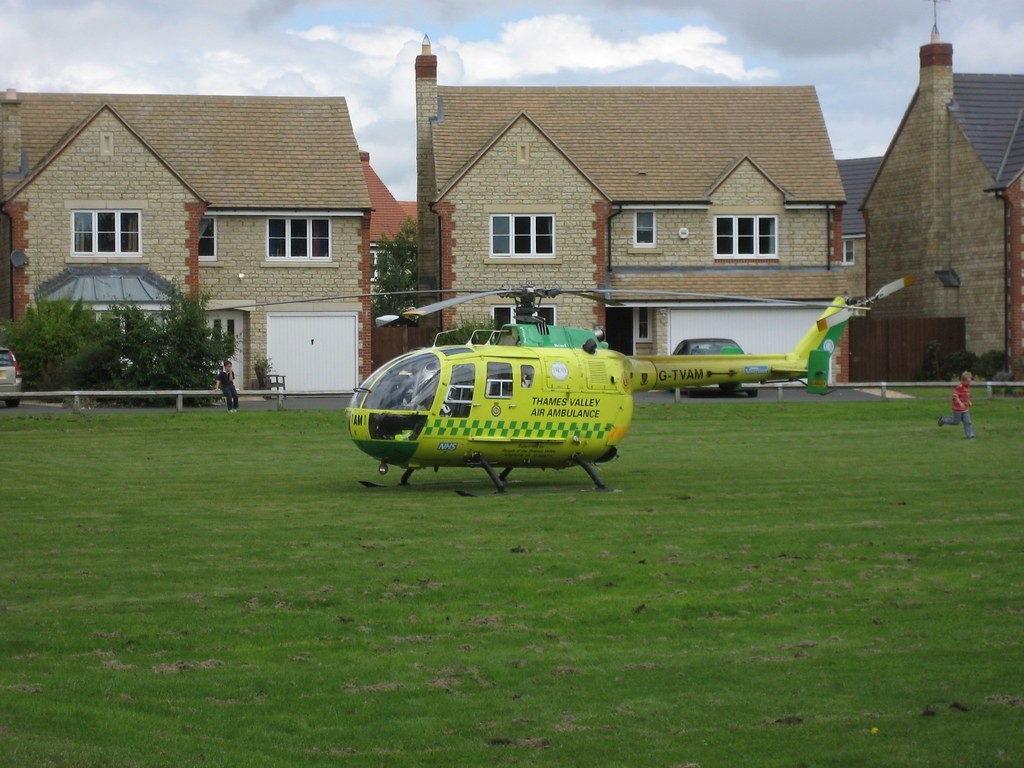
[937,416,945,427]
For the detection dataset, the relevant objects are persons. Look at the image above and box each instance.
[214,361,239,413]
[937,371,976,440]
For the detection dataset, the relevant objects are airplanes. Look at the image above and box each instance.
[201,275,914,497]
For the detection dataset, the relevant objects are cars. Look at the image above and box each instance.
[667,338,758,398]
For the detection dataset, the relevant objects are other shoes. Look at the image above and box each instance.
[967,436,976,441]
[228,408,238,414]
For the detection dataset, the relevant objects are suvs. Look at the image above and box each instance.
[0,346,23,407]
